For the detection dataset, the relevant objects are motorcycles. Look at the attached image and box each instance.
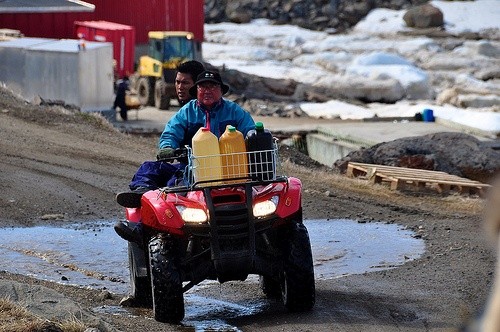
[125,144,316,323]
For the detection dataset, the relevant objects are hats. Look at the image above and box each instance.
[188,71,229,98]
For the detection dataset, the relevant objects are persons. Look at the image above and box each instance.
[113,76,129,118]
[115,70,258,249]
[175,60,205,108]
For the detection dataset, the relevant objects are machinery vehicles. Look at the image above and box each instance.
[128,30,203,110]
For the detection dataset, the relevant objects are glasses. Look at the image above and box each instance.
[196,84,221,92]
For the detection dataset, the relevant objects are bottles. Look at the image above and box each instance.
[245,122,275,181]
[192,127,226,187]
[218,125,249,183]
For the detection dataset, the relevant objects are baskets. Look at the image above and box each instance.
[184,136,279,190]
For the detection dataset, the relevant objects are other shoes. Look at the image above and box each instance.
[114,220,151,249]
[116,186,150,208]
